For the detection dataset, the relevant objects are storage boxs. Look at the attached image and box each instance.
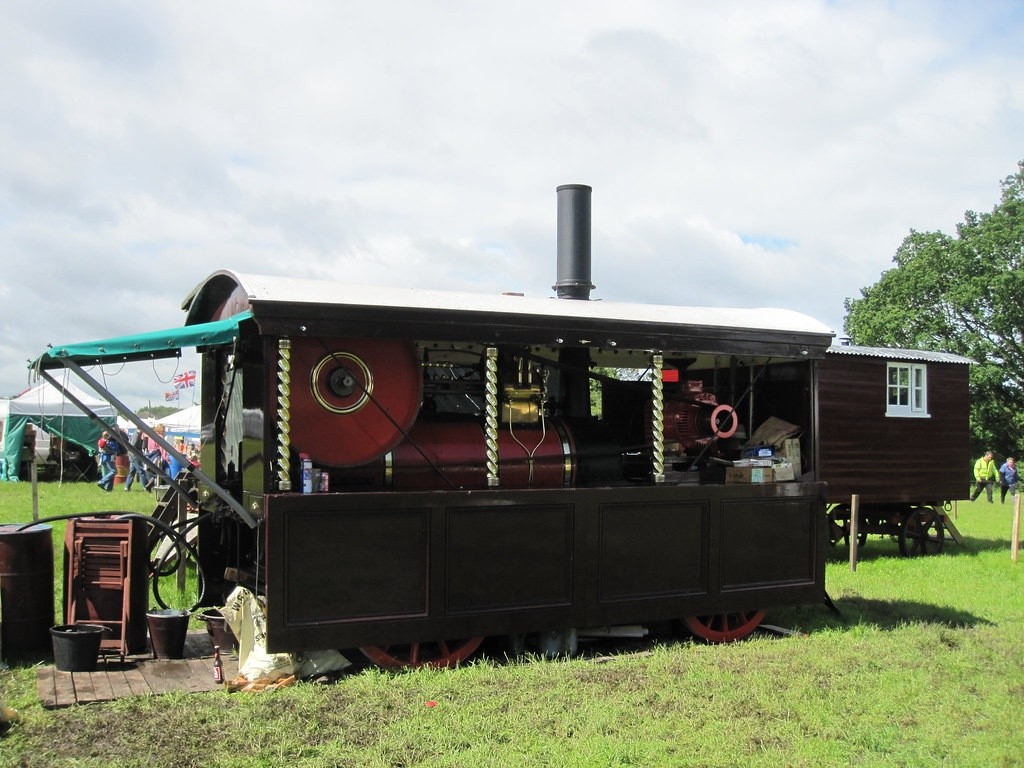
[780,438,802,479]
[719,467,773,483]
[773,466,795,482]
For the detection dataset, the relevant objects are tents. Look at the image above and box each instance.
[154,406,201,446]
[1,375,117,483]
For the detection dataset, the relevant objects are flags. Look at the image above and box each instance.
[173,370,196,389]
[166,390,180,402]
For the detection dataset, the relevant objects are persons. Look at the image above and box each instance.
[998,458,1024,504]
[970,451,1000,504]
[124,424,171,493]
[96,423,118,491]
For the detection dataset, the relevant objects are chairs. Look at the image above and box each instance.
[68,518,133,669]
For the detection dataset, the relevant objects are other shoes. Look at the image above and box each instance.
[97,483,106,490]
[124,486,131,492]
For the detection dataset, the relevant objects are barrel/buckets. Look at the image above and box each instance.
[196,609,238,655]
[155,484,172,503]
[0,523,54,653]
[114,454,129,485]
[49,621,105,673]
[168,453,188,481]
[145,606,193,659]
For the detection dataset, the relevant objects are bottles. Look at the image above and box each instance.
[301,458,312,493]
[213,646,225,684]
